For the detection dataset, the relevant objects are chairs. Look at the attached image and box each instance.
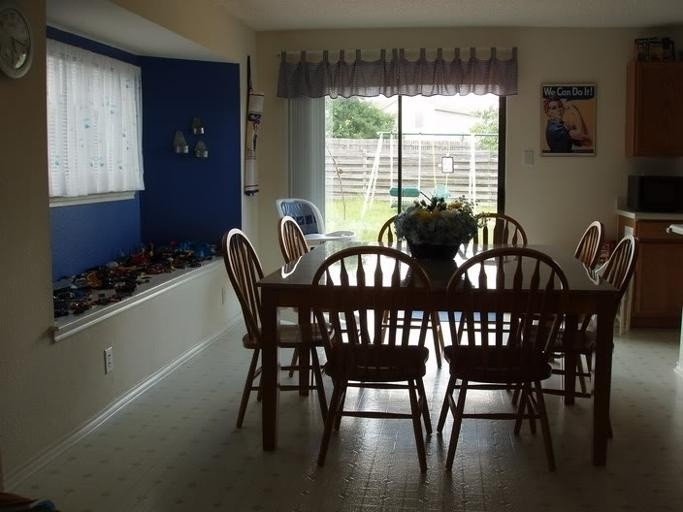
[311,245,433,473]
[377,216,445,351]
[457,212,528,346]
[437,247,568,471]
[279,217,358,378]
[512,235,640,406]
[220,227,336,429]
[505,221,604,381]
[276,198,356,247]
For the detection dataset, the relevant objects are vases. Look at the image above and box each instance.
[409,239,460,262]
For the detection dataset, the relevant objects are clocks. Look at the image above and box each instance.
[0,5,35,79]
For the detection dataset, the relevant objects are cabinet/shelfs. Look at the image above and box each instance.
[625,63,683,159]
[618,215,682,329]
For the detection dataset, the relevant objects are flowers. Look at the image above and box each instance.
[394,191,489,245]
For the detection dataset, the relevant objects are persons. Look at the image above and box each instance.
[544,98,590,153]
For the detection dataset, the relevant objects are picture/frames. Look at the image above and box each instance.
[539,81,598,157]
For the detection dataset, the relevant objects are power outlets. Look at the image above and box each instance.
[104,347,113,373]
